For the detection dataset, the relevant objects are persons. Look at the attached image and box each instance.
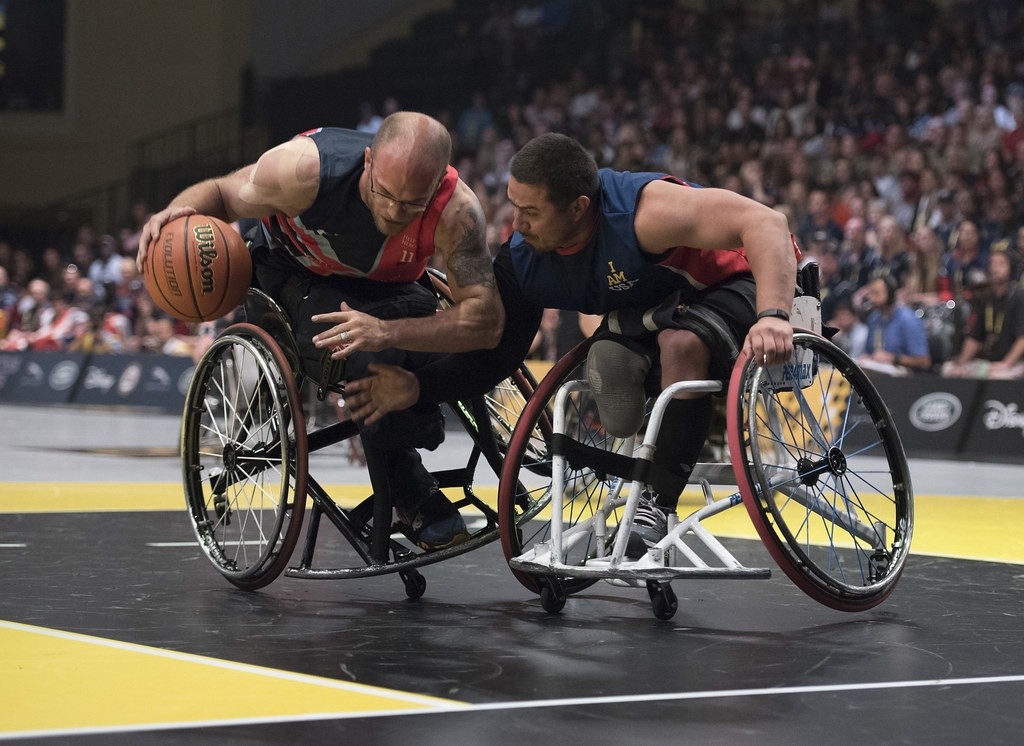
[0,0,1024,380]
[344,131,796,555]
[136,111,504,551]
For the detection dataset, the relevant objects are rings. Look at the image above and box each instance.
[341,331,349,341]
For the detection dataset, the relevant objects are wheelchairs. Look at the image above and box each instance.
[497,259,915,622]
[180,266,553,599]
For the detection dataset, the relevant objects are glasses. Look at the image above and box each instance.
[370,160,448,212]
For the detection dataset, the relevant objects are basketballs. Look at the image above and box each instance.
[142,213,253,325]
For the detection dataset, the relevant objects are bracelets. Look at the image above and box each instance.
[757,308,789,321]
[894,353,900,365]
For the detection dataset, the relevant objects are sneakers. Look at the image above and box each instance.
[383,447,469,550]
[618,488,676,565]
